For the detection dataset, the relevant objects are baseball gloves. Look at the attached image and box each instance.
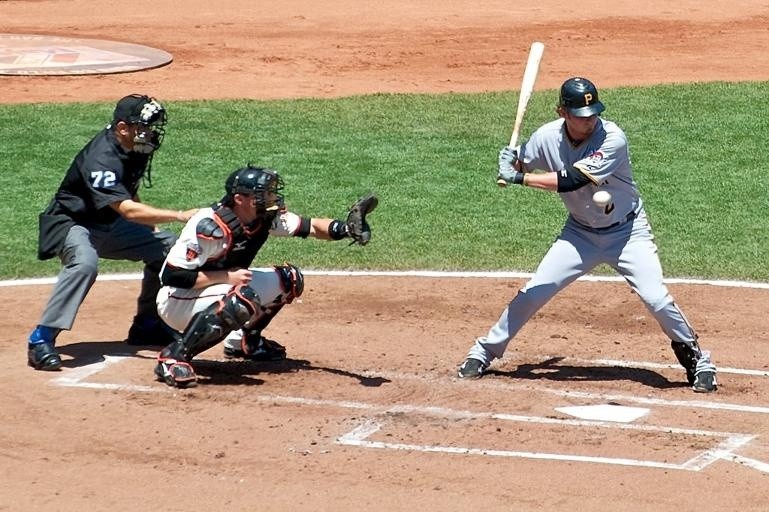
[344,194,379,246]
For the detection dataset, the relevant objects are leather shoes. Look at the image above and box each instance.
[130,318,183,348]
[27,337,61,370]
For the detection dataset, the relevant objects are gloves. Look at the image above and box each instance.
[498,146,517,165]
[497,161,518,184]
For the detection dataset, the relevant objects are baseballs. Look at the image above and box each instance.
[591,191,611,208]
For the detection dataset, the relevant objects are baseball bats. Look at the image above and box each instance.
[497,41,544,187]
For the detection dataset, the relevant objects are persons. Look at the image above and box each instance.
[459,78,718,394]
[27,92,199,373]
[153,165,380,389]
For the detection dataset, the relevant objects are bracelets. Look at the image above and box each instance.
[327,216,345,244]
[176,208,183,221]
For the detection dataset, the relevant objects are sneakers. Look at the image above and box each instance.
[223,339,288,361]
[691,368,718,394]
[153,348,199,388]
[459,356,484,378]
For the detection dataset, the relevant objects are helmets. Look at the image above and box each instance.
[559,77,604,118]
[225,166,285,217]
[114,94,167,154]
